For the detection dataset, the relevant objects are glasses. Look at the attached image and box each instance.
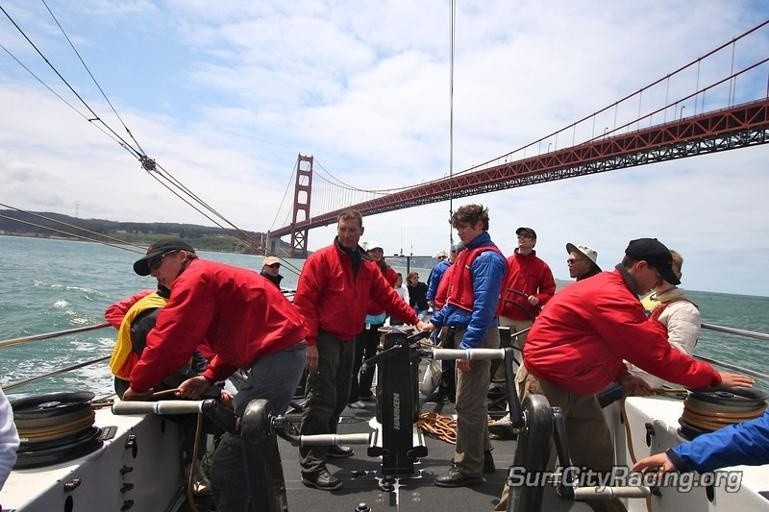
[143,249,178,276]
[517,233,534,238]
[265,264,280,269]
[438,255,446,260]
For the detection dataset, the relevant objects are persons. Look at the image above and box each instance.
[0,383,21,491]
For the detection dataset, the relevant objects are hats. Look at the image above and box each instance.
[364,240,384,252]
[624,237,682,285]
[515,227,537,240]
[133,237,196,277]
[565,241,603,275]
[261,256,281,268]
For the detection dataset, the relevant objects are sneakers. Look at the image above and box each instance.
[326,442,354,458]
[447,452,496,473]
[433,467,484,487]
[493,475,511,512]
[346,400,366,409]
[357,391,377,401]
[300,467,343,490]
